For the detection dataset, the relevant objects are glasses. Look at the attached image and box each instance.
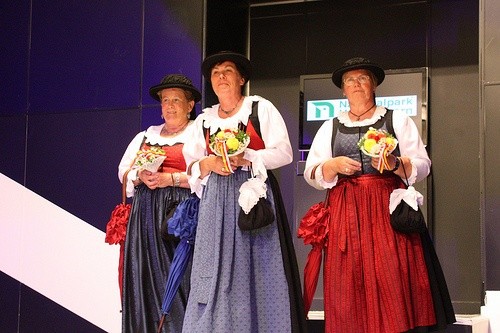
[344,73,370,85]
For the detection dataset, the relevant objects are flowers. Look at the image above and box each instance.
[358,127,398,157]
[209,127,250,157]
[133,142,166,173]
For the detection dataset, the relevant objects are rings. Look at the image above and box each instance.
[345,168,348,172]
[222,167,224,172]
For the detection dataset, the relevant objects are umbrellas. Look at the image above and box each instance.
[103,170,134,303]
[153,159,199,333]
[297,164,332,316]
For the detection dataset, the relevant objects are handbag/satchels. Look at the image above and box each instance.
[160,201,183,242]
[237,170,274,231]
[388,157,426,232]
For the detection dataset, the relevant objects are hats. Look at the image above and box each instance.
[331,57,386,88]
[149,74,202,104]
[200,50,253,88]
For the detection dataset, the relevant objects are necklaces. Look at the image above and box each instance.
[220,99,242,115]
[350,103,376,122]
[166,120,190,135]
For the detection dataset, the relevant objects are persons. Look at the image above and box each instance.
[182,50,305,333]
[302,54,459,333]
[116,74,209,332]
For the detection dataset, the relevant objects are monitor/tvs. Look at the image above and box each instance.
[299,67,429,150]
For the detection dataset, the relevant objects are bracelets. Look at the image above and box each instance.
[136,172,142,183]
[170,172,175,188]
[173,174,180,188]
[390,157,401,172]
